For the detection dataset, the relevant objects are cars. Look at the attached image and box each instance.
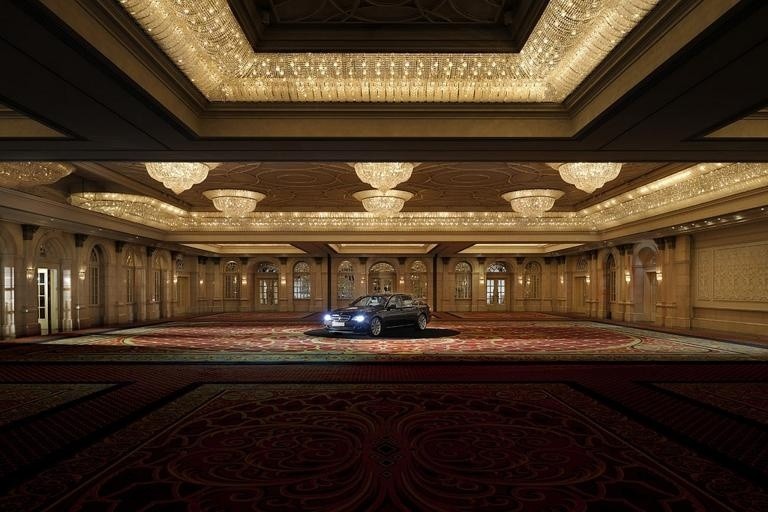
[320,293,432,336]
